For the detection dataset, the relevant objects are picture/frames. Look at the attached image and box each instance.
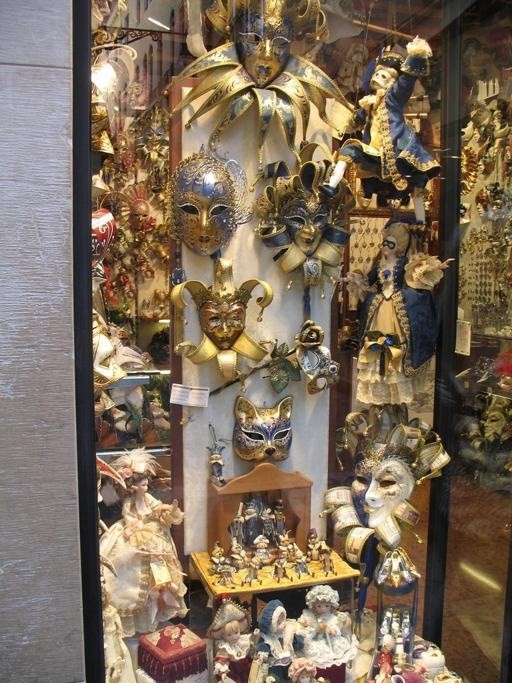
[343,210,431,321]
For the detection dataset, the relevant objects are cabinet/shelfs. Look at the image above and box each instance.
[188,463,361,629]
[93,369,173,463]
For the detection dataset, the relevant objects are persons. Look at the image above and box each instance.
[94,444,412,683]
[95,445,412,683]
[334,219,456,406]
[317,34,444,225]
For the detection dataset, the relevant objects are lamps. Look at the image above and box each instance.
[89,26,188,103]
